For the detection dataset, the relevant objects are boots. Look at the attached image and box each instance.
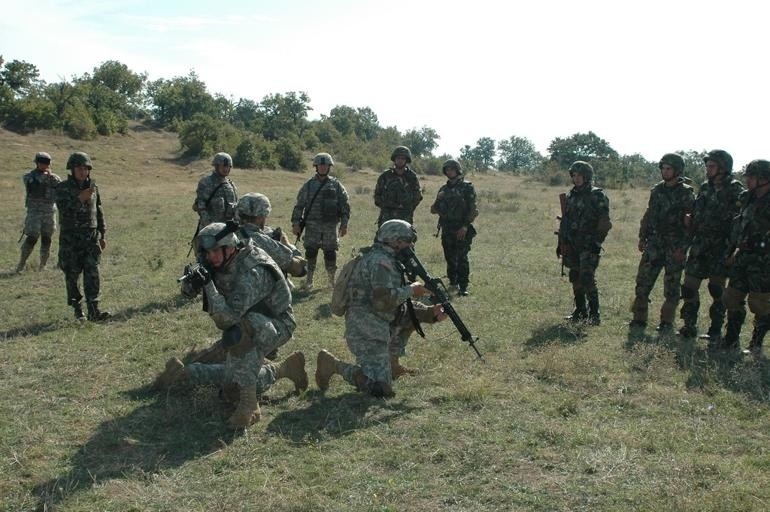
[15,255,29,274]
[70,299,88,323]
[315,349,340,394]
[286,348,309,394]
[87,300,112,322]
[564,297,588,322]
[38,255,54,273]
[226,381,263,428]
[585,300,602,326]
[391,347,424,380]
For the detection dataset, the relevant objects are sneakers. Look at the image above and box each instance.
[626,318,649,328]
[458,285,470,296]
[721,334,741,350]
[448,277,458,286]
[324,274,338,289]
[655,322,673,332]
[297,279,315,292]
[744,337,762,353]
[676,326,697,336]
[700,329,721,340]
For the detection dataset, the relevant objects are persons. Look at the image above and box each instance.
[54,151,112,322]
[374,145,423,225]
[629,152,696,331]
[315,219,449,398]
[706,159,769,357]
[13,151,63,274]
[152,190,311,389]
[555,160,612,324]
[674,148,744,341]
[194,151,240,230]
[291,152,351,289]
[179,221,311,430]
[430,159,480,296]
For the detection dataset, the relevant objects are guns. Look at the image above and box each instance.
[178,247,215,312]
[554,193,567,277]
[396,246,483,359]
[434,181,472,238]
[187,217,202,258]
[294,218,305,245]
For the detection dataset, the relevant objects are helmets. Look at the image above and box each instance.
[235,193,272,217]
[195,221,241,251]
[702,150,734,174]
[440,159,464,176]
[311,152,335,167]
[33,151,53,165]
[568,161,594,181]
[65,150,94,170]
[658,152,686,172]
[741,159,770,179]
[389,146,413,164]
[211,152,233,168]
[376,218,419,246]
